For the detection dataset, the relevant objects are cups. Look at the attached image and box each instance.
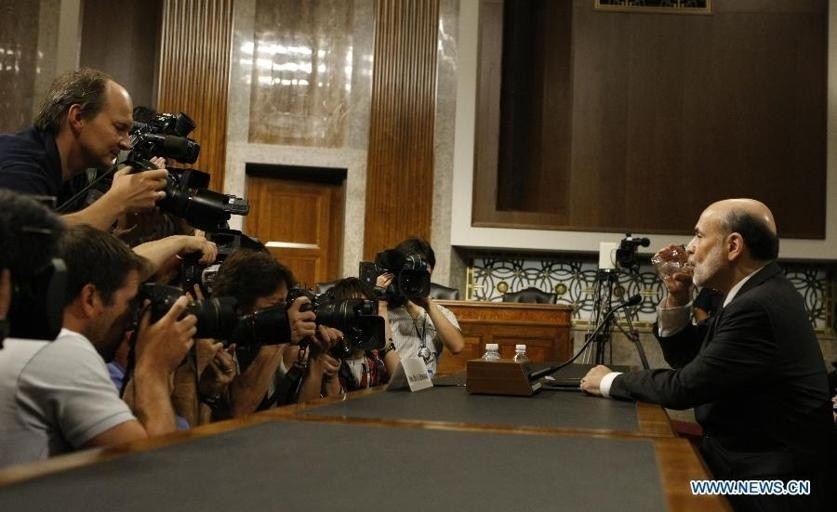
[653,248,693,277]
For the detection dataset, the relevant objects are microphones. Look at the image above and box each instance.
[529,293,642,383]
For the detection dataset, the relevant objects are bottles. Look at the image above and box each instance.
[480,344,526,362]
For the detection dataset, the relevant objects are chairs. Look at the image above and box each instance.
[428,282,561,303]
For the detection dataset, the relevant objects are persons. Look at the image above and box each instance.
[580,198,837,512]
[0,66,466,471]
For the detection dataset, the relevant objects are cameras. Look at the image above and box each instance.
[0,257,66,340]
[215,303,291,350]
[285,288,365,346]
[328,337,353,358]
[127,158,230,234]
[137,283,239,341]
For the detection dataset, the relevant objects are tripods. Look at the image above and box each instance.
[581,274,650,371]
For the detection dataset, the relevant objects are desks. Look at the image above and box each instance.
[0,360,736,512]
